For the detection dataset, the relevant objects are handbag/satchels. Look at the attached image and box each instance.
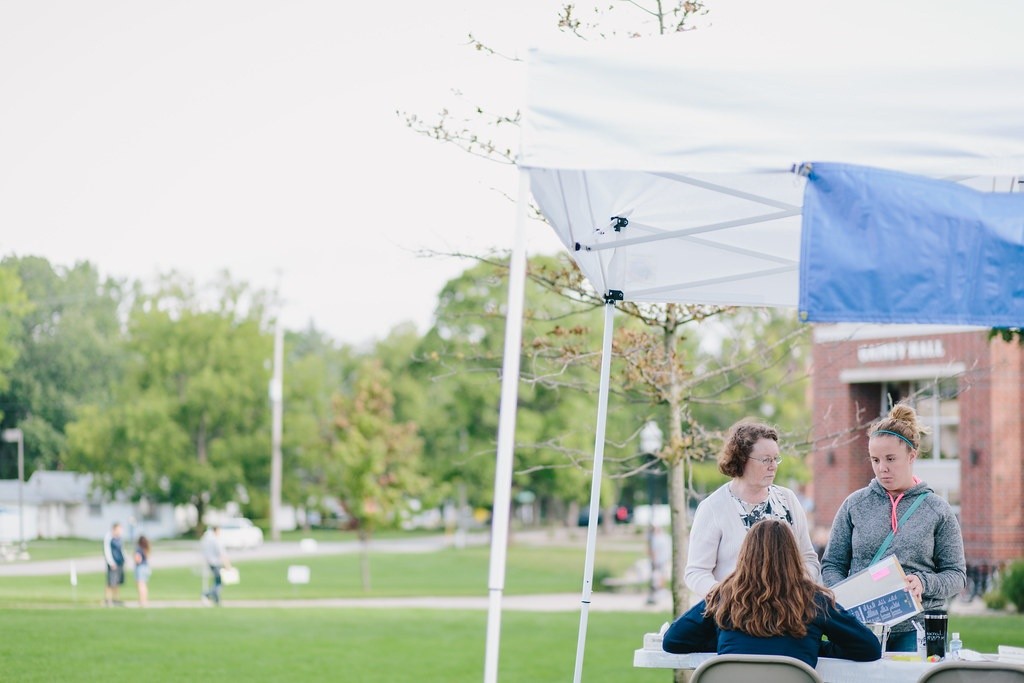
[220,566,240,585]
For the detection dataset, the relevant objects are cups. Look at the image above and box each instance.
[917,631,926,658]
[924,610,947,662]
[863,623,891,659]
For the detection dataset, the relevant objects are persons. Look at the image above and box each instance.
[821,406,966,655]
[132,535,153,606]
[662,520,881,677]
[683,421,821,600]
[102,523,125,608]
[201,526,230,605]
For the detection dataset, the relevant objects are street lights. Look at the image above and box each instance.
[640,421,663,605]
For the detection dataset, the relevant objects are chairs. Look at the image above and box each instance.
[691,654,1024,683]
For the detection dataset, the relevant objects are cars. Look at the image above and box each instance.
[200,517,263,549]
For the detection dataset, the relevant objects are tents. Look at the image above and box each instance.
[482,48,1024,683]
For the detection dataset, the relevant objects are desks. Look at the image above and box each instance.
[634,648,1023,682]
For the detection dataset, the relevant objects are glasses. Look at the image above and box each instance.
[749,456,782,465]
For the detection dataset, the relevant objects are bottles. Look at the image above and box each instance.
[949,633,962,661]
